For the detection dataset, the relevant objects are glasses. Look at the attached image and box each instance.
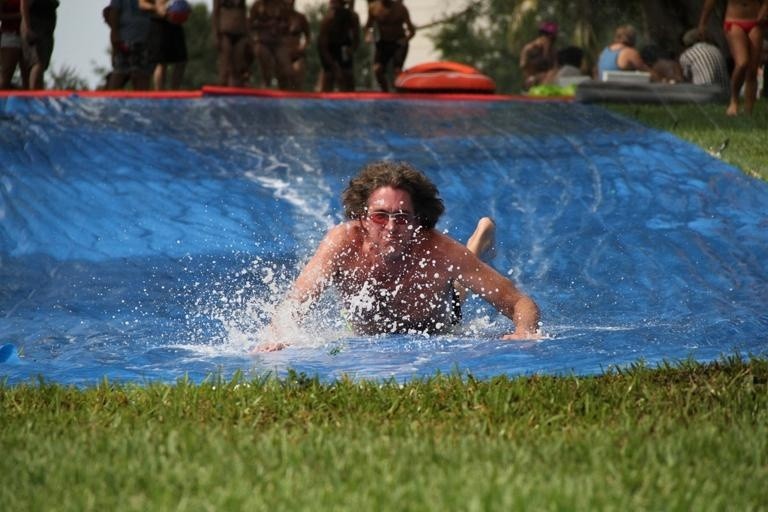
[361,210,421,225]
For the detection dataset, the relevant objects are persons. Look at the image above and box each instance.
[253,163,539,351]
